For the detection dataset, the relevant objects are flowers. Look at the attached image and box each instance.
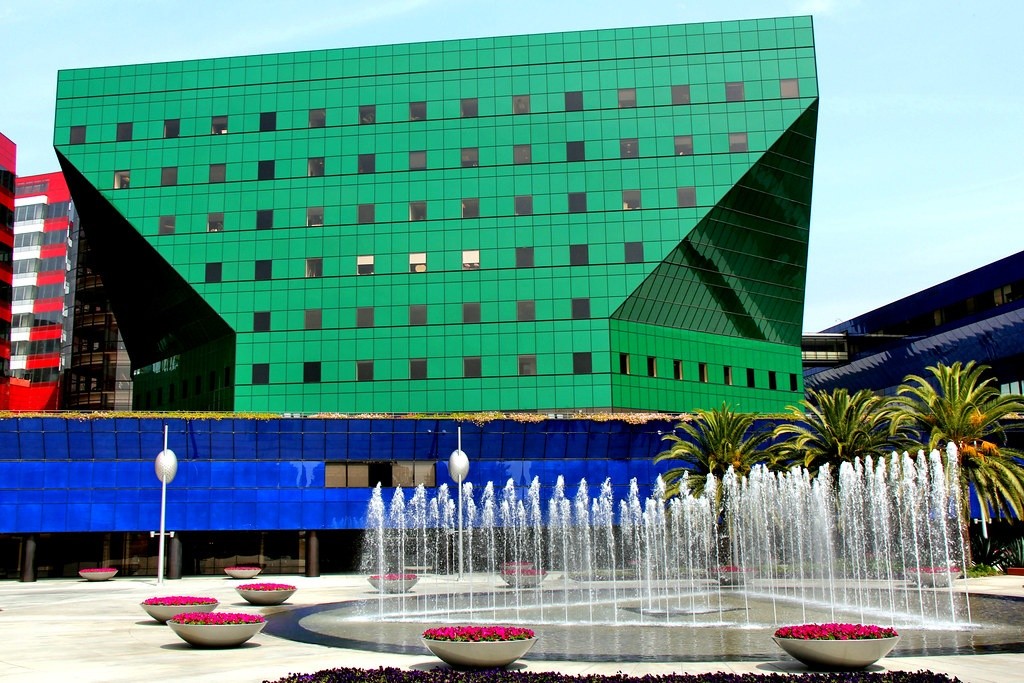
[707,566,757,573]
[368,573,417,580]
[903,565,961,573]
[499,562,534,566]
[169,612,265,628]
[79,567,117,573]
[235,583,297,591]
[421,626,534,643]
[141,595,219,606]
[772,622,897,641]
[223,566,260,570]
[500,568,547,576]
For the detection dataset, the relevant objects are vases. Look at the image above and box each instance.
[223,570,261,579]
[906,572,960,587]
[500,565,534,570]
[235,590,296,604]
[499,574,546,588]
[78,572,118,580]
[166,619,267,648]
[420,636,539,669]
[710,572,756,585]
[139,604,219,624]
[366,578,420,593]
[770,636,900,670]
